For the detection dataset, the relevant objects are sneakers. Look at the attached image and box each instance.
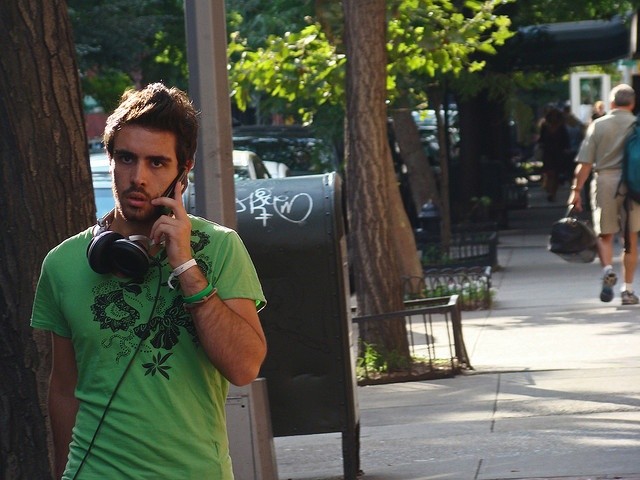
[599,268,617,302]
[621,289,640,305]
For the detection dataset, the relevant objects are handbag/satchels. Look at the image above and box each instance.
[547,203,598,264]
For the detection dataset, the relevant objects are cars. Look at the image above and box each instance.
[232,131,340,175]
[230,149,270,182]
[256,158,292,177]
[89,153,189,223]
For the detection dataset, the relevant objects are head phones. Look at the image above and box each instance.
[86,207,175,277]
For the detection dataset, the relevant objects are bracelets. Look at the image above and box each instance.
[183,286,219,311]
[167,259,197,288]
[183,283,214,302]
[570,186,583,193]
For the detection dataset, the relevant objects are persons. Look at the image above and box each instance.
[567,84,639,305]
[537,103,559,190]
[32,86,267,479]
[589,101,606,124]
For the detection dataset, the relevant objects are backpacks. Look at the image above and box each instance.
[613,113,640,254]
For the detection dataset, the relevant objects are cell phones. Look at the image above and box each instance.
[158,170,188,214]
